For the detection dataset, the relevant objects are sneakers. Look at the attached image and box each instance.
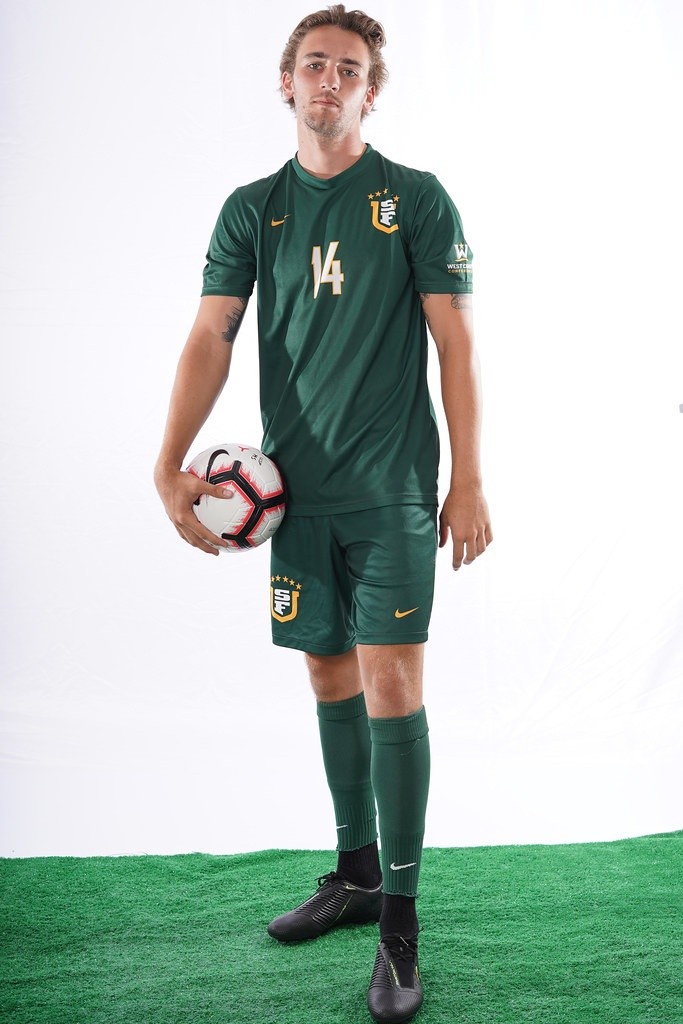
[366,930,424,1022]
[267,870,384,943]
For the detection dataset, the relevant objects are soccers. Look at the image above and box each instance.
[180,442,286,553]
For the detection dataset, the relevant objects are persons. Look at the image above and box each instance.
[151,2,497,1020]
